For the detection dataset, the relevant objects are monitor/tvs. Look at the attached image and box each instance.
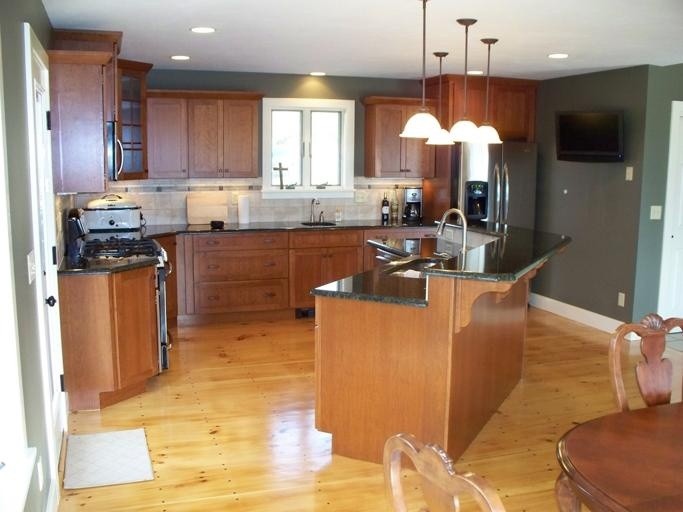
[556,110,624,162]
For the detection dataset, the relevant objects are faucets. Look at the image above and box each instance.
[310,198,320,222]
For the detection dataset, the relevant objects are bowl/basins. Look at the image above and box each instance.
[209,221,225,229]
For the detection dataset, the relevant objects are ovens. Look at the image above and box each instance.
[156,263,171,373]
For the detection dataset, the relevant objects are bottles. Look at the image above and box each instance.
[381,189,398,220]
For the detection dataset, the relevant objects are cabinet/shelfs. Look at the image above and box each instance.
[45,31,152,195]
[364,227,438,267]
[184,233,289,315]
[420,74,539,144]
[152,236,177,320]
[144,89,263,180]
[360,94,435,180]
[288,230,362,309]
[110,266,159,390]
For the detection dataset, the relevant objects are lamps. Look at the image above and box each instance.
[399,0,504,146]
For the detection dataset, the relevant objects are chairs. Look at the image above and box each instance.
[610,313,682,413]
[384,431,503,512]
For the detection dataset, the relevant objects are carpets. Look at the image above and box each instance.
[63,427,153,491]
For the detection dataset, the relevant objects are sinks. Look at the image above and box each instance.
[301,222,336,226]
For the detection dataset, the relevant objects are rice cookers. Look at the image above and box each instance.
[82,193,142,232]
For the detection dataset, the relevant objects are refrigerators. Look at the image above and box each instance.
[456,141,538,229]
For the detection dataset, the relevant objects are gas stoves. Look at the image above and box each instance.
[81,239,168,270]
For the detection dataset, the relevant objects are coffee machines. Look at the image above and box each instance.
[401,186,423,221]
